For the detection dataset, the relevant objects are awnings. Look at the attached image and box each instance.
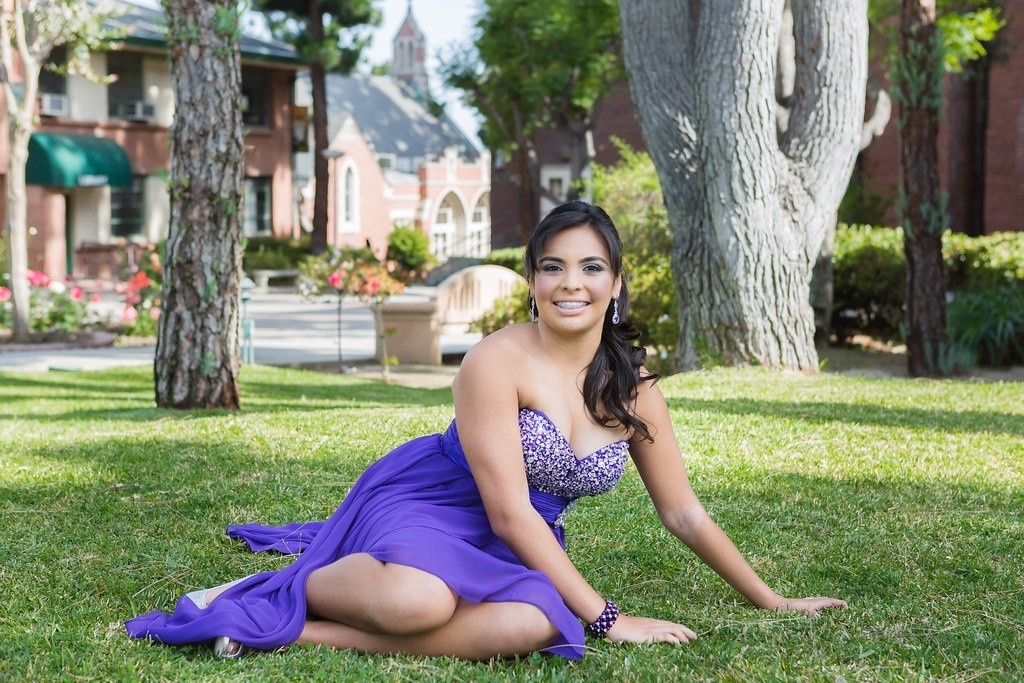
[25,134,132,188]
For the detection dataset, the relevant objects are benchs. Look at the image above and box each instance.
[252,269,299,288]
[370,264,530,364]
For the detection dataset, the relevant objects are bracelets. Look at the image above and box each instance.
[781,598,786,608]
[589,601,620,636]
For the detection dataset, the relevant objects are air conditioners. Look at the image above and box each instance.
[128,101,154,122]
[38,93,69,117]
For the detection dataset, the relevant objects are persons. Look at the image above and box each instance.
[123,202,847,663]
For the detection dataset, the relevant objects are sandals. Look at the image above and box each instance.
[185,573,255,609]
[214,636,244,661]
